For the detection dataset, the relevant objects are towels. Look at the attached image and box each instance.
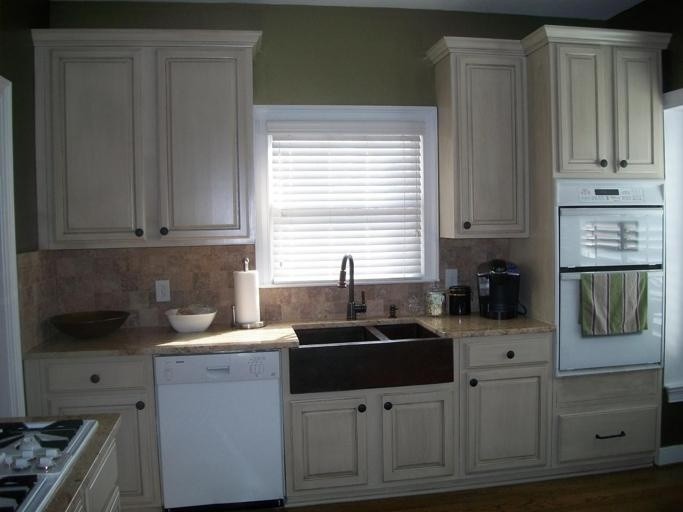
[579,272,648,335]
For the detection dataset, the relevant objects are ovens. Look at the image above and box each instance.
[552,178,665,379]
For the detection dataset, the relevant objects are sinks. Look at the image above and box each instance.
[373,317,443,343]
[292,320,381,349]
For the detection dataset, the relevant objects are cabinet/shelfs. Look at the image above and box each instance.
[558,404,661,461]
[25,355,161,511]
[290,391,452,504]
[32,28,265,251]
[462,339,547,480]
[524,25,674,178]
[427,35,530,240]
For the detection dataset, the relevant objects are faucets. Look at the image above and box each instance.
[337,255,366,320]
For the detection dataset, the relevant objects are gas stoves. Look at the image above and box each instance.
[0,418,98,512]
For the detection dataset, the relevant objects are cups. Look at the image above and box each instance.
[448,286,472,315]
[424,286,447,317]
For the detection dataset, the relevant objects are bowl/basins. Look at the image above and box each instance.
[51,311,131,340]
[165,307,218,332]
